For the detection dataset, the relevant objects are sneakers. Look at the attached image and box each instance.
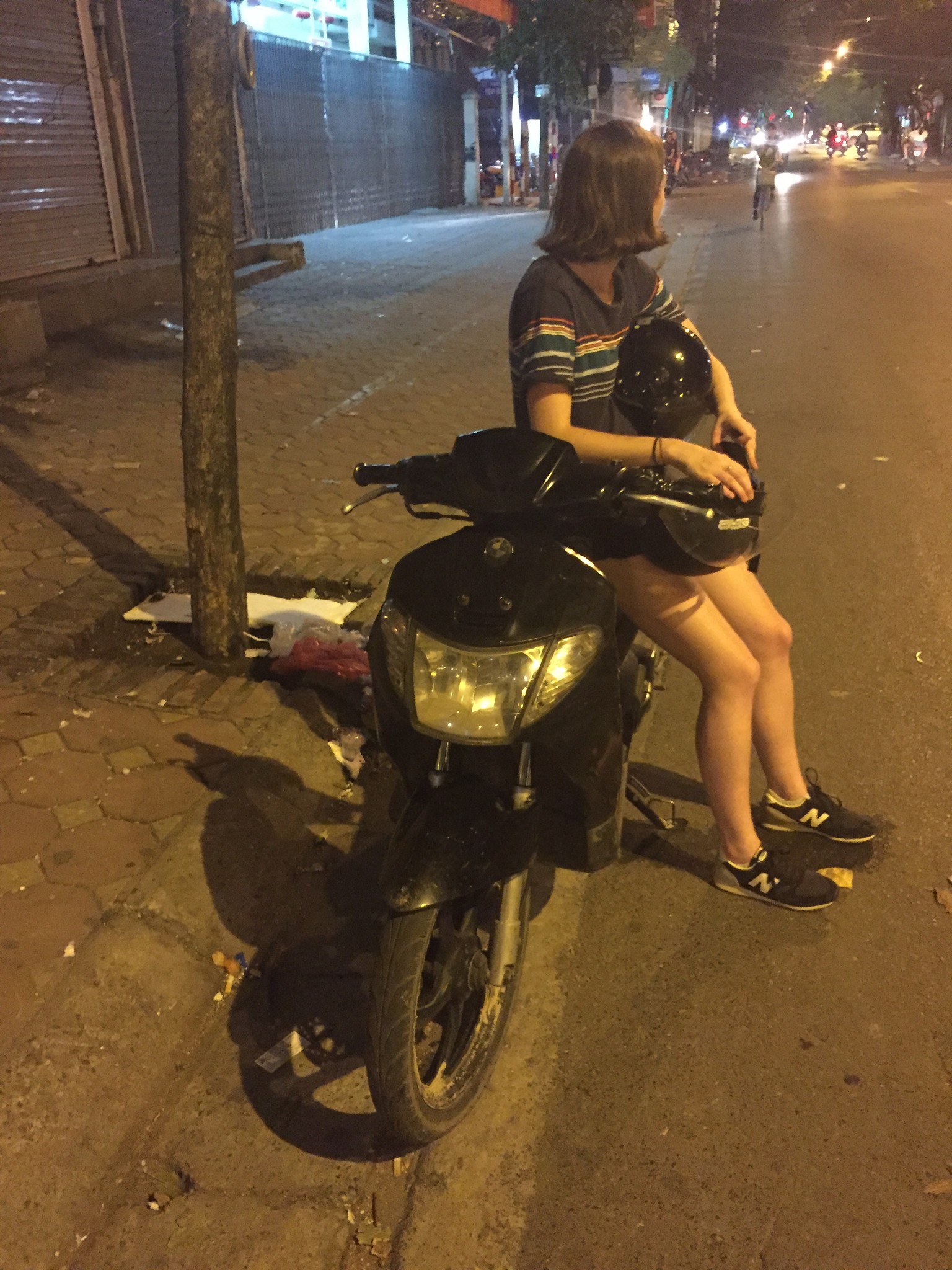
[712,840,839,911]
[759,767,875,843]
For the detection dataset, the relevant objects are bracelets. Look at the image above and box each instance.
[651,435,663,468]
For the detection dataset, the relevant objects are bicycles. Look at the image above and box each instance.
[748,158,783,232]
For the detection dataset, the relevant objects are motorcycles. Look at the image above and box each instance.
[855,142,868,159]
[903,142,924,172]
[359,425,767,1148]
[664,157,677,193]
[826,140,850,159]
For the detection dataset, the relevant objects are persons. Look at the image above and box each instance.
[505,120,877,912]
[661,118,929,221]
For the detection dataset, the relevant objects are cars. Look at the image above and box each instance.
[844,122,883,145]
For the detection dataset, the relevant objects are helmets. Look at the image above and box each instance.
[622,443,757,577]
[612,313,713,413]
